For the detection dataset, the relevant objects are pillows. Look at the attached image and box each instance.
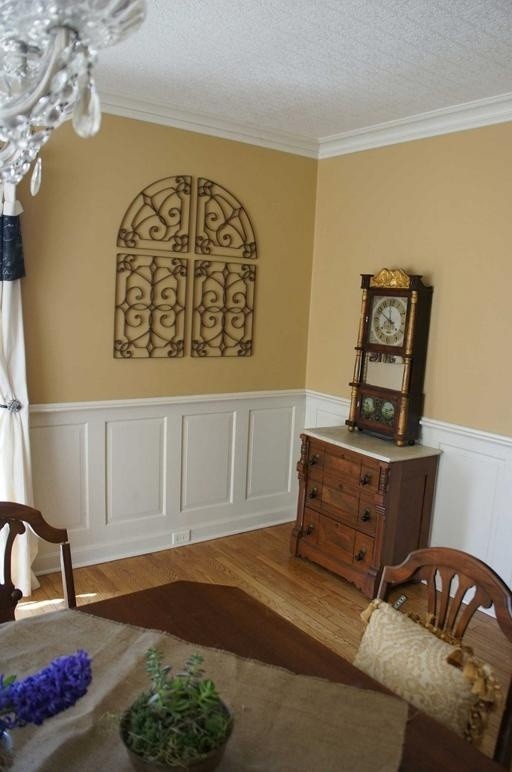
[356,597,502,748]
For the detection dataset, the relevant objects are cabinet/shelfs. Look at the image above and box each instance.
[290,425,443,598]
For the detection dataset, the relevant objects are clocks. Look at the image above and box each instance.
[345,271,435,445]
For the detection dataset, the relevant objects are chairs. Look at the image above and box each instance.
[355,547,512,767]
[0,500,76,622]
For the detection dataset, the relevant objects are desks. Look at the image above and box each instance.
[0,579,505,772]
